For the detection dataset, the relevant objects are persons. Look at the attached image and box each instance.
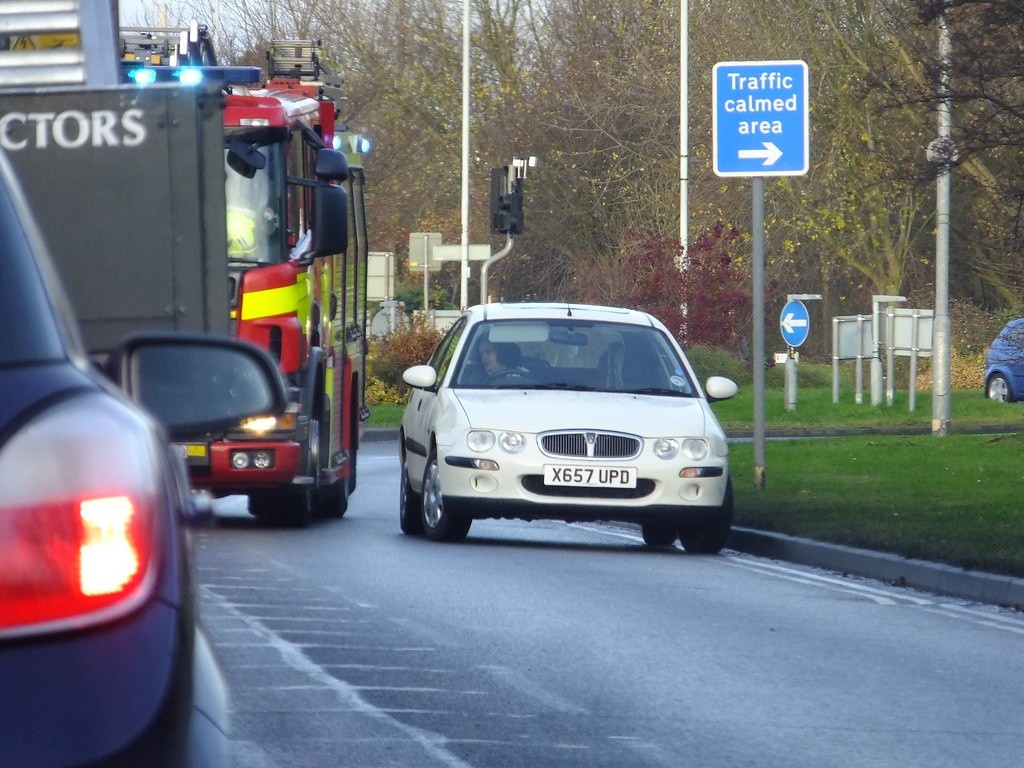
[463,332,540,388]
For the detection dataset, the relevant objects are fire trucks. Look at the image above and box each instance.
[0,2,371,527]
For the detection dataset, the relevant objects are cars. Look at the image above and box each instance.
[0,149,290,768]
[984,317,1024,405]
[399,299,739,554]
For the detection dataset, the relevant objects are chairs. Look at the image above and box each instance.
[523,361,553,385]
[595,342,647,389]
